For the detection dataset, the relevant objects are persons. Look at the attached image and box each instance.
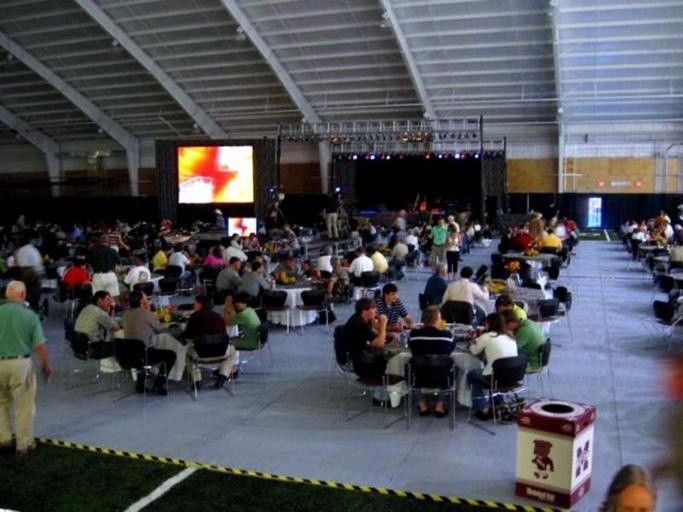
[0,279,51,455]
[500,211,578,252]
[429,210,474,280]
[2,218,421,396]
[325,195,340,238]
[407,263,546,419]
[622,210,674,247]
[599,463,658,512]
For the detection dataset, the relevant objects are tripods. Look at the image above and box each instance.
[266,199,287,224]
[334,200,354,233]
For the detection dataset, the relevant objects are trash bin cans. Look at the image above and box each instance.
[515,396,596,509]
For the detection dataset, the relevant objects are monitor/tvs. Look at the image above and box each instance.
[228,217,258,238]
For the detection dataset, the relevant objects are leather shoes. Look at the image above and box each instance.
[152,385,167,395]
[191,380,203,390]
[214,379,225,389]
[372,397,390,408]
[136,385,149,392]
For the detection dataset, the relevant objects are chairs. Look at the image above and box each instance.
[1,224,683,435]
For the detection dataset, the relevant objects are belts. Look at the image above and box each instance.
[4,355,30,359]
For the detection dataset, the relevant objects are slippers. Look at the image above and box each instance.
[419,408,430,416]
[435,408,448,418]
